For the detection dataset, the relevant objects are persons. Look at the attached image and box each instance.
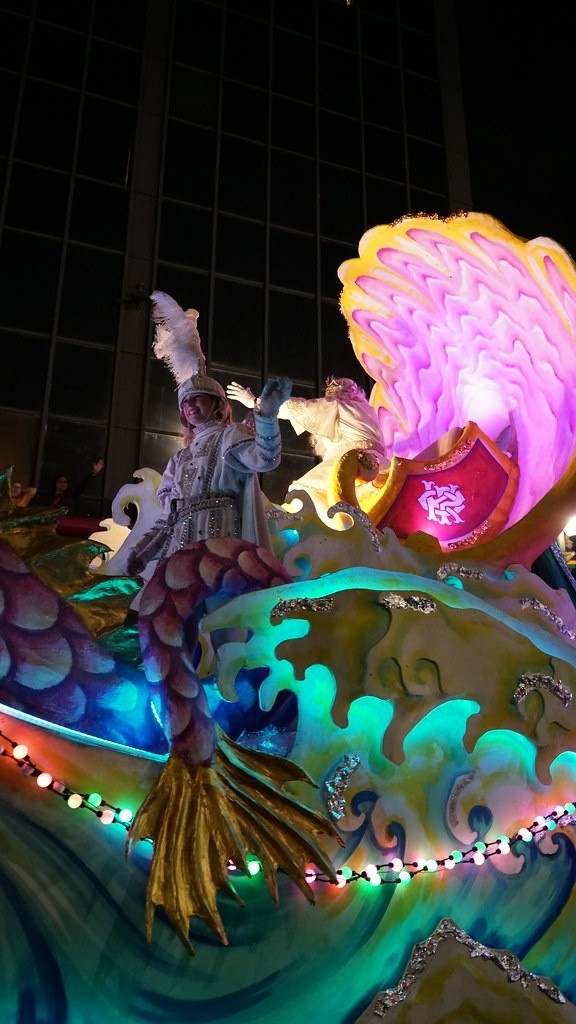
[10,480,37,507]
[39,458,105,507]
[123,376,293,577]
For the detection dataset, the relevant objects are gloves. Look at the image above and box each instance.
[125,547,148,576]
[261,376,293,418]
[226,381,261,409]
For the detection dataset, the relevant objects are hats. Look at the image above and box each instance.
[150,291,227,411]
